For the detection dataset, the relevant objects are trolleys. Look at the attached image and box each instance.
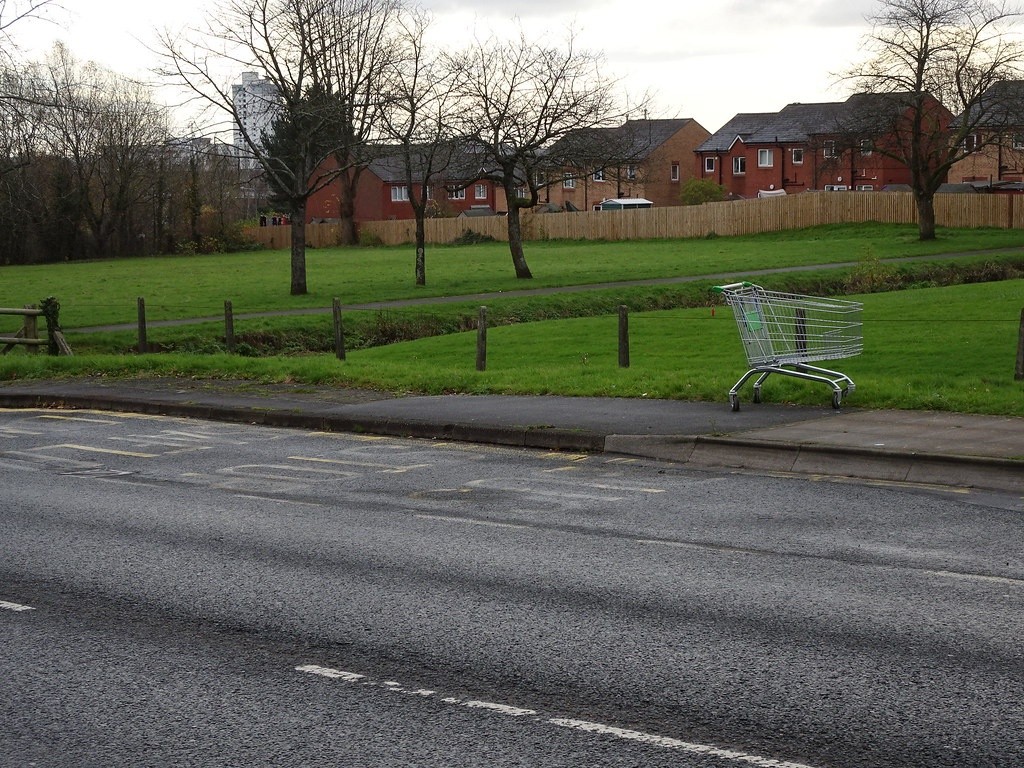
[711,281,865,411]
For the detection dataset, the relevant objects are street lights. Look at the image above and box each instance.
[241,187,258,220]
